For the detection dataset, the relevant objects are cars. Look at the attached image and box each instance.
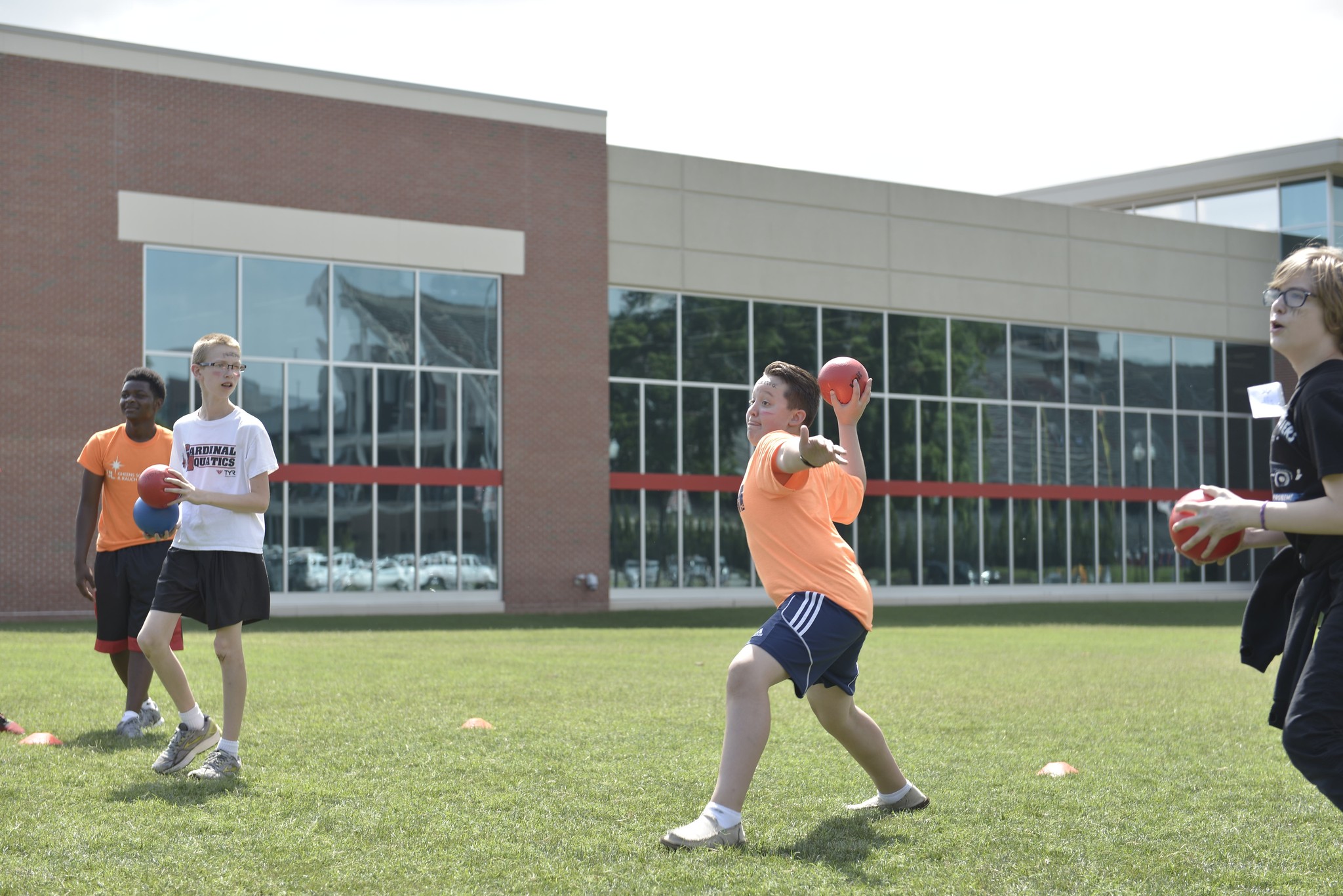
[263,544,496,590]
[668,556,728,586]
[913,559,1001,584]
[625,560,659,587]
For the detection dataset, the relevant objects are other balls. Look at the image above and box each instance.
[818,356,869,406]
[1169,489,1246,561]
[137,464,182,509]
[133,496,179,536]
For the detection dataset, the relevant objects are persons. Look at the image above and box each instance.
[74,368,183,739]
[1173,246,1343,813]
[661,360,930,854]
[136,333,279,781]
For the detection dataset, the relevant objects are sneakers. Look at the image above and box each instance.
[139,701,164,729]
[187,748,242,781]
[845,784,931,813]
[116,717,144,739]
[151,714,222,776]
[661,812,745,852]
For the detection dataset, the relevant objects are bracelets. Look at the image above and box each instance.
[800,456,816,468]
[1260,500,1270,531]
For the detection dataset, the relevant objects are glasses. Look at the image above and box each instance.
[199,362,246,373]
[1262,288,1322,308]
